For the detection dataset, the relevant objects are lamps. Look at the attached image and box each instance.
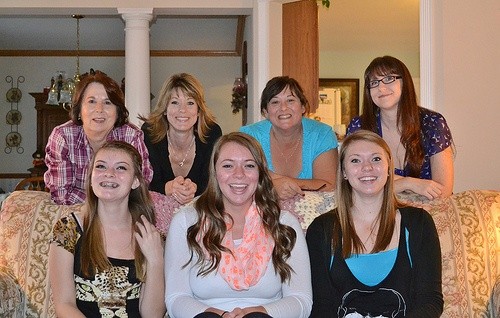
[45,14,86,112]
[233,78,248,109]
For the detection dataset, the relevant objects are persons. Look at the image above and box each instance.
[239,76,339,202]
[304,130,444,318]
[347,55,458,199]
[136,73,222,205]
[44,70,154,205]
[51,141,165,318]
[164,132,313,318]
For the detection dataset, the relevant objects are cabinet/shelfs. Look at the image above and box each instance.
[28,92,72,155]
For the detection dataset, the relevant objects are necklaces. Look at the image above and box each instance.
[172,136,195,167]
[275,137,299,163]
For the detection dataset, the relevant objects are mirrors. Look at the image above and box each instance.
[309,78,359,141]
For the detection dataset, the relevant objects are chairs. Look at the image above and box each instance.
[15,176,51,192]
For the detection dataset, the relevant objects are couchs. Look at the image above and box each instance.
[0,191,499,318]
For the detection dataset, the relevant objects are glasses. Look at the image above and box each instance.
[367,75,401,89]
